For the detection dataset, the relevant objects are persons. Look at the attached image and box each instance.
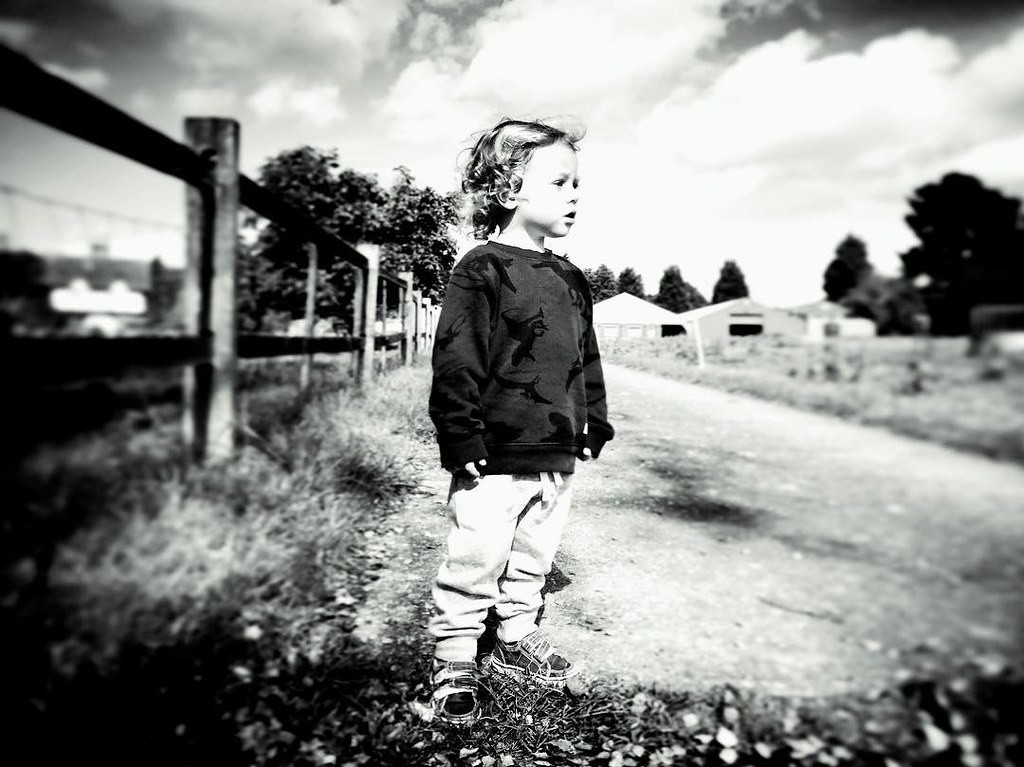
[428,117,614,721]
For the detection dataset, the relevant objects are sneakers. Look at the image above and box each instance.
[491,630,576,680]
[430,658,479,724]
